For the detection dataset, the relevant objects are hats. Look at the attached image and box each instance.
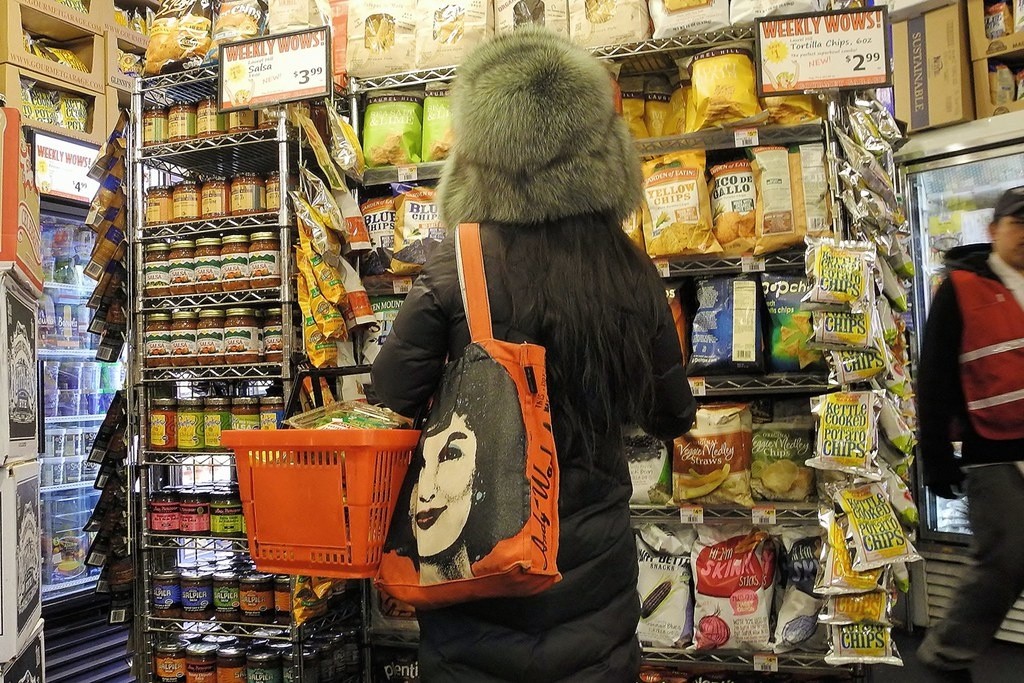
[434,26,646,233]
[995,185,1024,218]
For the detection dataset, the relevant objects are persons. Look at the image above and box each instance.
[911,187,1023,683]
[364,38,694,683]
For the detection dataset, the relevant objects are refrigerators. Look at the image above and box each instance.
[894,110,1023,642]
[22,123,128,683]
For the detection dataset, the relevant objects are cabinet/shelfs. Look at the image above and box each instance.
[91,30,844,683]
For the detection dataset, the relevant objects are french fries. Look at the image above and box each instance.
[678,466,750,503]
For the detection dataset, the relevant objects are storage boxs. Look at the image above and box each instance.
[104,0,161,41]
[104,29,147,92]
[972,49,1024,120]
[0,270,39,467]
[0,107,42,299]
[0,63,107,146]
[0,460,43,661]
[0,0,106,94]
[967,0,1024,62]
[892,3,976,136]
[0,618,46,683]
[106,86,158,140]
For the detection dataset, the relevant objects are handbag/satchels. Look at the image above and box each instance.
[370,221,563,608]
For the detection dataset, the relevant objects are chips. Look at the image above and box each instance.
[706,86,748,122]
[752,459,811,495]
[649,219,710,253]
[765,95,815,125]
[429,126,454,160]
[371,133,405,166]
[716,212,755,241]
[781,316,822,362]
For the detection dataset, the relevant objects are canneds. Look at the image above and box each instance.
[139,98,291,683]
